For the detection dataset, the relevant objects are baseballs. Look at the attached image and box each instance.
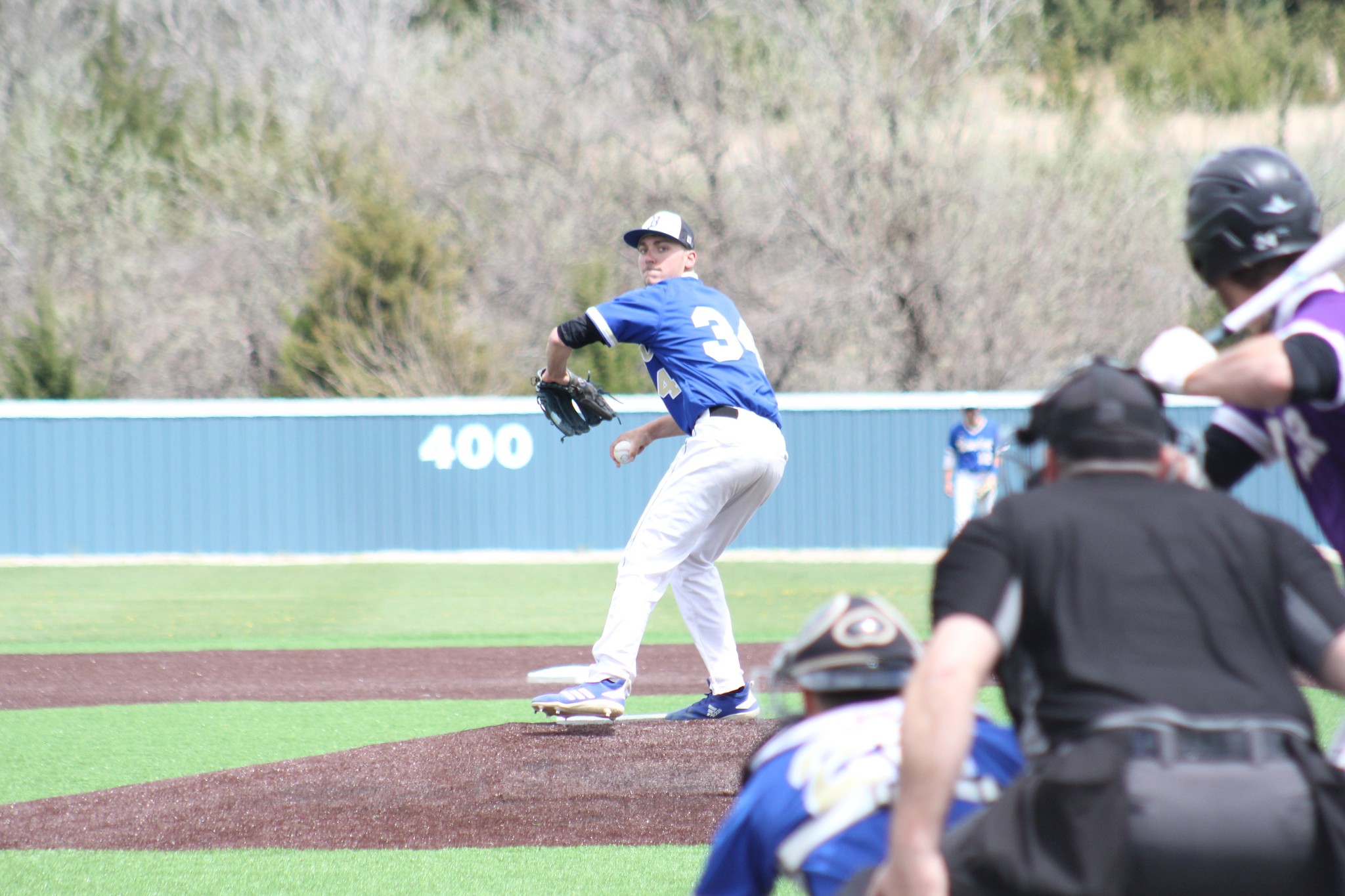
[613,442,634,465]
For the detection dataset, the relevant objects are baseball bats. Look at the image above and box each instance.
[1199,219,1345,344]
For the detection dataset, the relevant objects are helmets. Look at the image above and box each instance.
[1179,144,1323,285]
[769,587,917,696]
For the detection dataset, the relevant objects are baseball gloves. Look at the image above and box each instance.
[536,365,618,437]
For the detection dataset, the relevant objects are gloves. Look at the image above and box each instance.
[1136,324,1220,396]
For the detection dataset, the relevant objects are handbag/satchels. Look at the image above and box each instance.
[960,741,1134,894]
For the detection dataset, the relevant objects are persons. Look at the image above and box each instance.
[693,587,1030,896]
[530,208,791,723]
[1137,143,1345,565]
[872,353,1345,896]
[943,408,1010,542]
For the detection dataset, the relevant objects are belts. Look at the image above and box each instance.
[708,405,739,420]
[1126,729,1293,762]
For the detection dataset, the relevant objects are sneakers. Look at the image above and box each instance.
[663,677,761,721]
[530,679,625,721]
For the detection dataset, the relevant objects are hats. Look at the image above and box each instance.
[960,391,980,411]
[622,211,695,250]
[1014,355,1170,462]
